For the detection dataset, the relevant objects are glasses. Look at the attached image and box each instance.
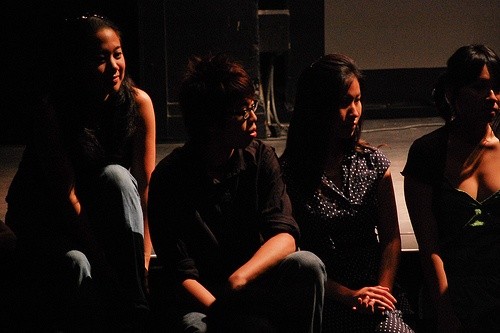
[227,94,261,121]
[66,12,104,28]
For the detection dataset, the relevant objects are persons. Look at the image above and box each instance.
[146,52,327,333]
[277,54,420,333]
[400,43,500,333]
[5,14,155,333]
